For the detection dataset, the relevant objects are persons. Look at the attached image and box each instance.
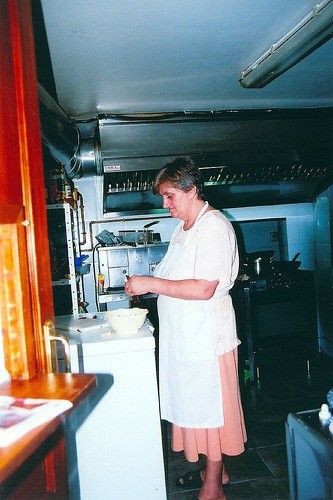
[124,157,248,500]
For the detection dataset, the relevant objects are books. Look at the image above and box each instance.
[0,395,74,449]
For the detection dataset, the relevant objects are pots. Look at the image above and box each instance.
[270,260,302,273]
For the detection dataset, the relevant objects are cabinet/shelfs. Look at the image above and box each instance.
[45,203,85,315]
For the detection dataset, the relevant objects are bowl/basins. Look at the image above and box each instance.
[108,308,148,337]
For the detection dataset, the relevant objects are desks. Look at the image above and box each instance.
[0,373,99,500]
[55,311,167,500]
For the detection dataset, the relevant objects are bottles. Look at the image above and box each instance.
[44,162,78,211]
[98,271,105,295]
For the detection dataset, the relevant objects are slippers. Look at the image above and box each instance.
[175,470,229,489]
